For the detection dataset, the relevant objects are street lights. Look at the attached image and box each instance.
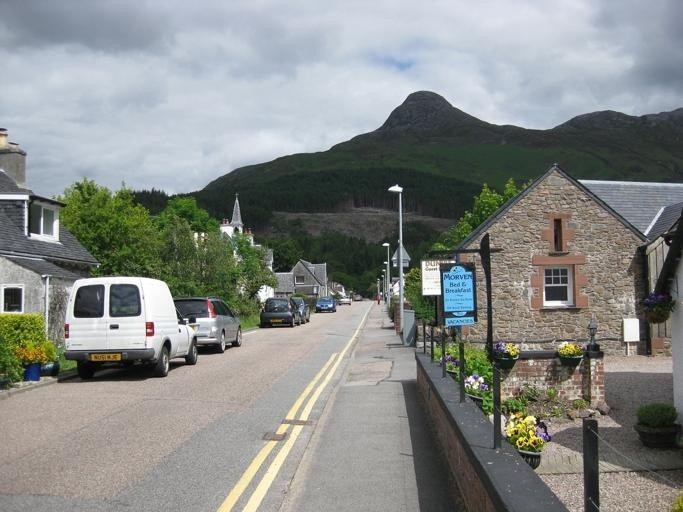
[388,184,404,334]
[375,242,390,310]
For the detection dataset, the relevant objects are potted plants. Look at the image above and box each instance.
[634,401,683,454]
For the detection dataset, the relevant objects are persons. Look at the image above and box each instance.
[377,292,381,304]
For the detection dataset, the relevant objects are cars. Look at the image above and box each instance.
[259,296,302,327]
[352,294,362,302]
[291,297,310,324]
[315,297,336,312]
[336,294,352,306]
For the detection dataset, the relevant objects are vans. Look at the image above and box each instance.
[171,296,242,352]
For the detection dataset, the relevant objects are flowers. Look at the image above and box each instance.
[634,291,679,318]
[14,342,50,366]
[433,326,588,470]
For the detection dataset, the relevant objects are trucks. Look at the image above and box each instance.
[62,276,198,377]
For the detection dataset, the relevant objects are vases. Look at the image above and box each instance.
[642,309,671,325]
[23,363,41,382]
